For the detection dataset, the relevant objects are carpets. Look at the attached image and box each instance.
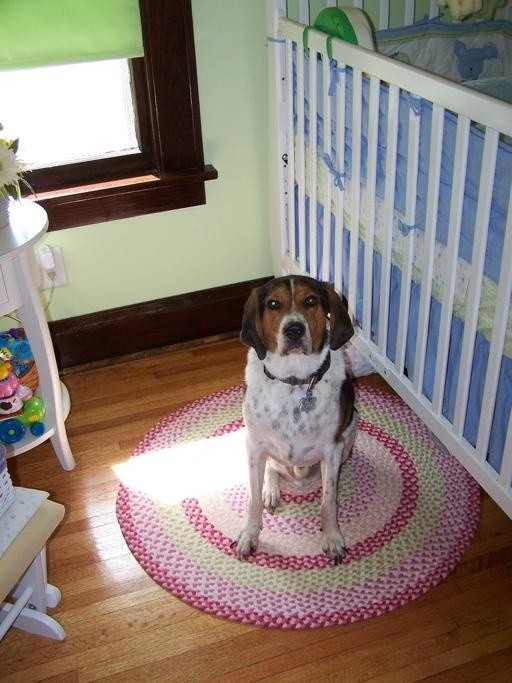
[115,382,481,630]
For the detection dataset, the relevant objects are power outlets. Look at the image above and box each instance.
[34,245,68,294]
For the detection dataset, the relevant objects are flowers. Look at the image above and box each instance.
[0,121,38,199]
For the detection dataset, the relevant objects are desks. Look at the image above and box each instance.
[0,197,77,473]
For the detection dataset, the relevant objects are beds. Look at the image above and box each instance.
[271,0,511,547]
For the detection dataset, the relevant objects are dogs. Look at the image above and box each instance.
[231,273,365,567]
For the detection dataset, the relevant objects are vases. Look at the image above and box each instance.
[0,198,10,230]
